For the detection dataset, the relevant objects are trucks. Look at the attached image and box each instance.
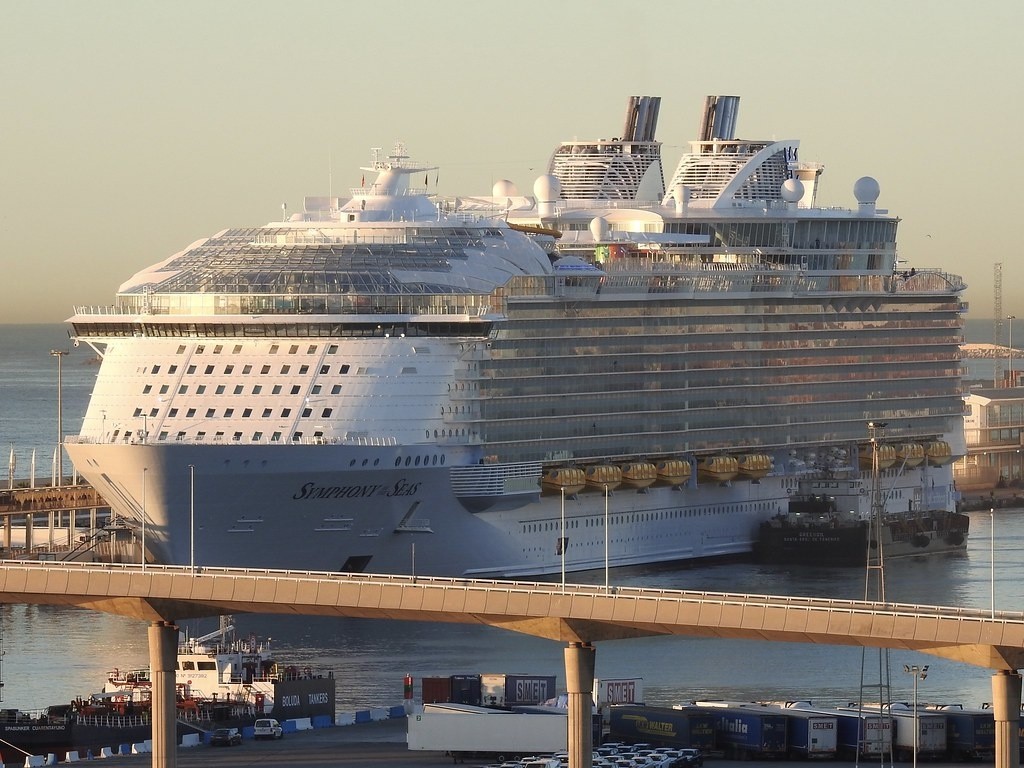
[610,703,1024,754]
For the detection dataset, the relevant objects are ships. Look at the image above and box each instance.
[59,95,971,579]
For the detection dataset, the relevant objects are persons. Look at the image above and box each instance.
[809,493,826,503]
[903,268,915,280]
[13,493,91,511]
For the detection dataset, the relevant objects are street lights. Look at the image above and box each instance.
[144,467,148,573]
[903,664,929,768]
[559,487,566,595]
[990,509,994,621]
[188,464,195,574]
[603,483,610,597]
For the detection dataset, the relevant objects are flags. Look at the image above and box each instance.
[425,175,427,186]
[361,176,365,186]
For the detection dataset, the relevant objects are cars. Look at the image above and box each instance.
[502,744,703,768]
[210,728,243,747]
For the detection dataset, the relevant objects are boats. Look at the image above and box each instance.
[0,615,335,768]
[49,346,69,356]
[749,479,972,578]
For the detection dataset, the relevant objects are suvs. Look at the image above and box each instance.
[254,719,284,739]
